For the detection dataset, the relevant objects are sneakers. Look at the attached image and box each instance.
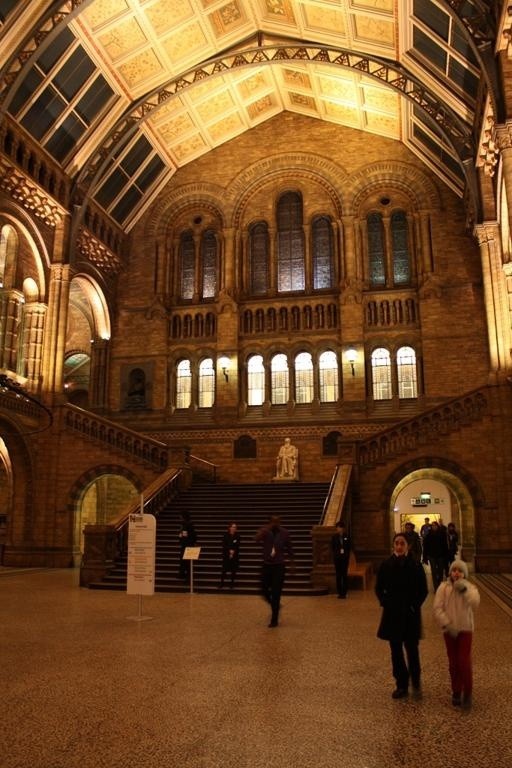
[392,676,473,707]
[178,570,347,628]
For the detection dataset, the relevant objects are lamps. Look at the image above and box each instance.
[218,353,229,383]
[345,345,358,376]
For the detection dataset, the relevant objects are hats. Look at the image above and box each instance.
[449,559,469,579]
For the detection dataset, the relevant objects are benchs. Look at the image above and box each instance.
[347,550,375,590]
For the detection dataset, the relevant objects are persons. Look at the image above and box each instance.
[404,518,459,594]
[218,520,240,587]
[330,522,351,599]
[373,533,429,699]
[176,510,197,586]
[278,437,296,477]
[431,560,481,711]
[255,514,295,628]
